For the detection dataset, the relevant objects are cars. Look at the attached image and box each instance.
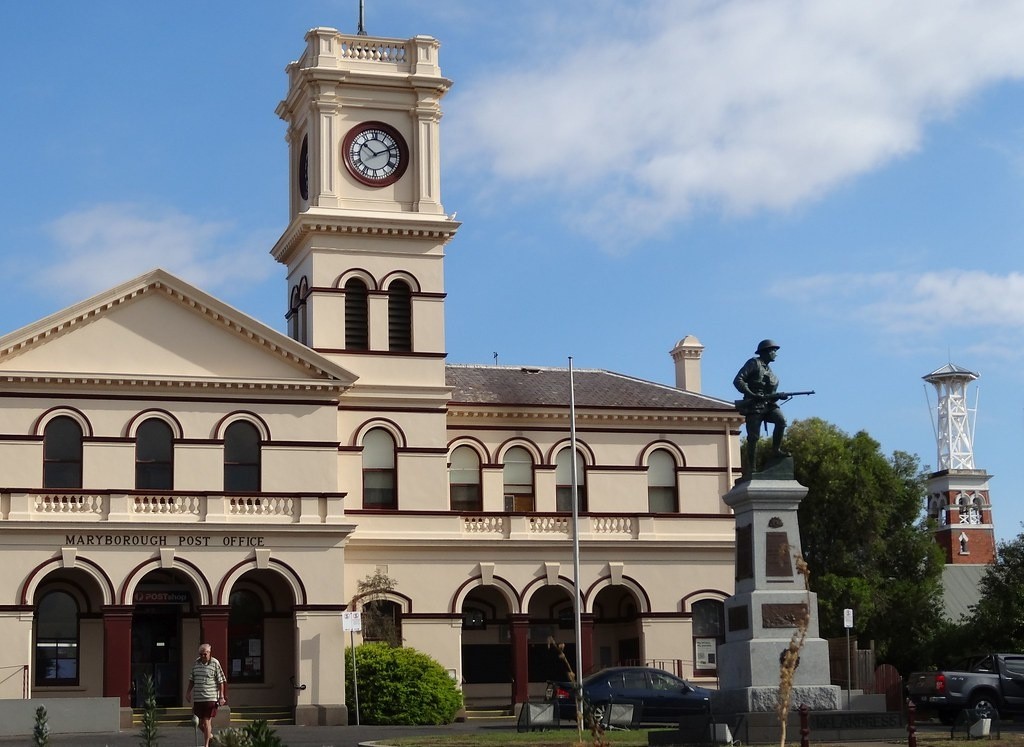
[545,666,741,727]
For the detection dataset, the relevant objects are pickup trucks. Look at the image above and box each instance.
[906,653,1024,728]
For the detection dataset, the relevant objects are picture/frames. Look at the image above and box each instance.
[499,624,553,643]
[693,636,717,670]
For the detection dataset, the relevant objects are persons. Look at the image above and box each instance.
[186,644,227,747]
[734,340,792,477]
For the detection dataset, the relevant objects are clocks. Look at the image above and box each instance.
[342,121,409,187]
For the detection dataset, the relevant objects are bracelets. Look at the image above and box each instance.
[219,697,224,699]
